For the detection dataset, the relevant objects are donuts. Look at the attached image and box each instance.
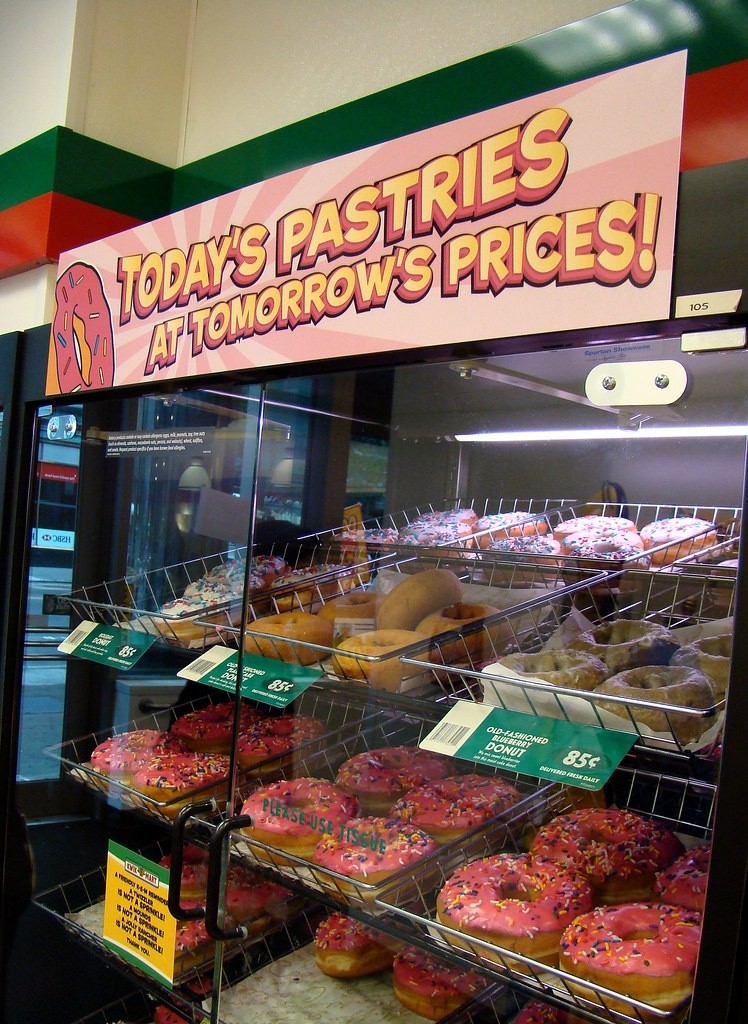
[91,511,739,1024]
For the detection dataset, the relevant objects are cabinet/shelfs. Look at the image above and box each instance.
[20,497,743,1024]
